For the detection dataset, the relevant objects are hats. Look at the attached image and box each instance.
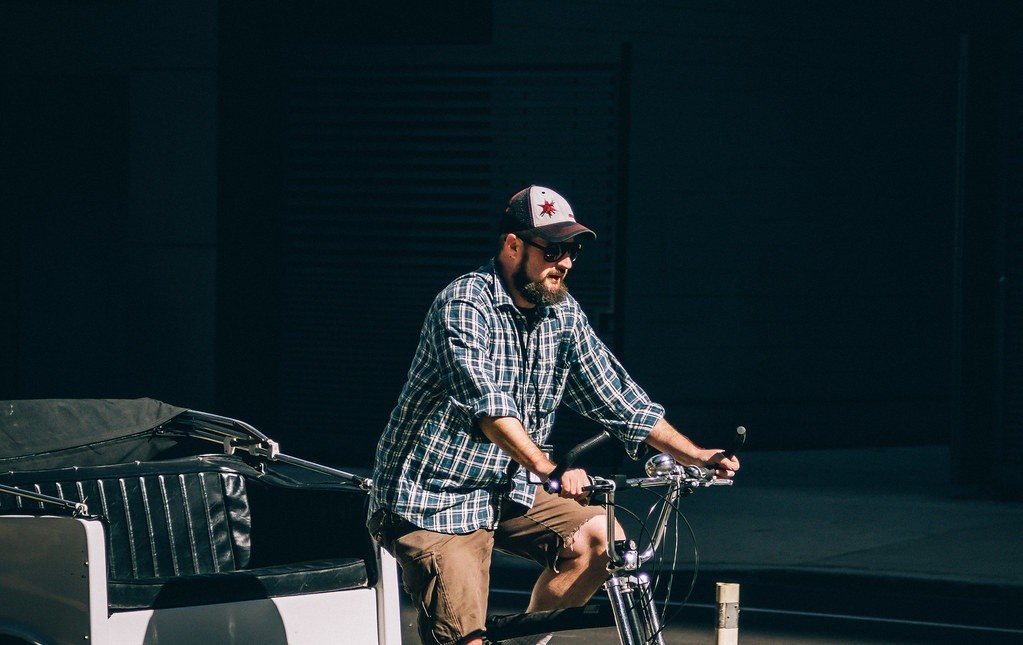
[499,184,597,243]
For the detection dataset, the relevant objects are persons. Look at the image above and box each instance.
[366,184,740,645]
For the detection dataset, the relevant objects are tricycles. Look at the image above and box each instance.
[0,395,747,645]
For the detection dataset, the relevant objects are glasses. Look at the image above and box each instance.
[504,235,583,263]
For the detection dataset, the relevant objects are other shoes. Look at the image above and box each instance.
[485,614,503,645]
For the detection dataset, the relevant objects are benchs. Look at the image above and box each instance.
[0,395,403,645]
